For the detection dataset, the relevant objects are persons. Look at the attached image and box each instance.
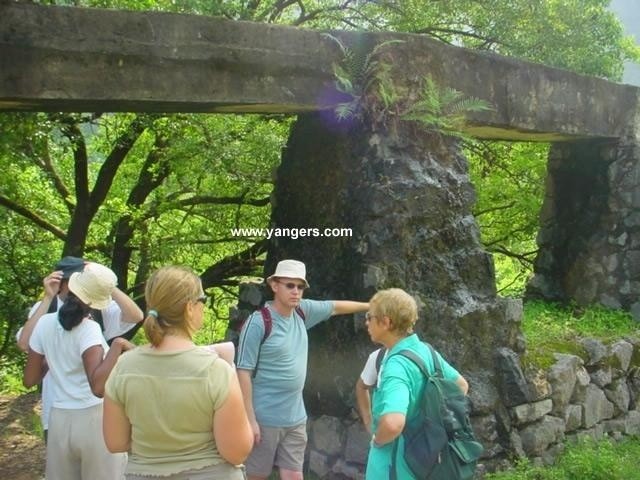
[355,288,469,480]
[236,259,370,480]
[103,266,254,480]
[15,256,144,480]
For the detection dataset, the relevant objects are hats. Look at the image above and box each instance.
[267,259,310,288]
[56,256,117,310]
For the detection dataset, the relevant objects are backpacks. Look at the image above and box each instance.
[397,342,484,480]
[234,306,305,378]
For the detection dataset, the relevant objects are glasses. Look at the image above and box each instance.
[274,279,305,289]
[366,312,377,322]
[194,296,207,304]
